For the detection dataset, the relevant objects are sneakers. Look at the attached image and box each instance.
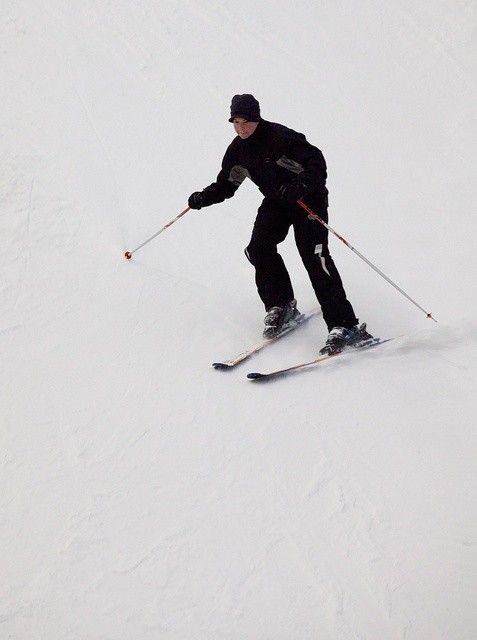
[326,324,359,346]
[262,303,288,325]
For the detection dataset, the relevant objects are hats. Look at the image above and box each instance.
[228,94,264,122]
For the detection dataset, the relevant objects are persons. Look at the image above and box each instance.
[186,94,361,354]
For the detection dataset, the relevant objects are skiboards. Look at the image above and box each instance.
[212,308,392,379]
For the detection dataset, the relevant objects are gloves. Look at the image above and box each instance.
[188,191,212,209]
[275,179,312,202]
